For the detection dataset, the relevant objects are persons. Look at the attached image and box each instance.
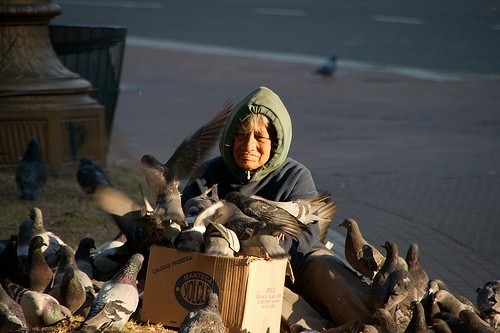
[182,86,377,333]
[312,55,338,77]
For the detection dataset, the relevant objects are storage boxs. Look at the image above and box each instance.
[140,244,295,333]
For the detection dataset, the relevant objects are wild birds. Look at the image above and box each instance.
[140,98,336,261]
[337,216,500,333]
[0,207,144,333]
[179,288,227,333]
